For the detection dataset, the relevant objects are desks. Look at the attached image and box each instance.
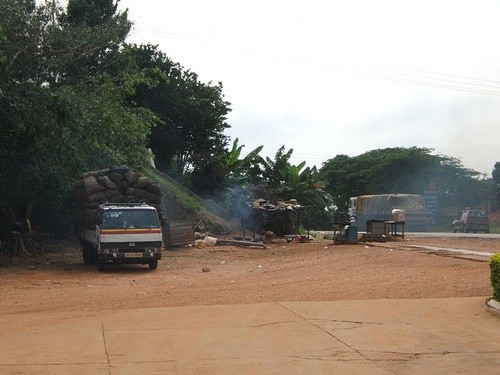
[383,221,405,239]
[333,224,344,238]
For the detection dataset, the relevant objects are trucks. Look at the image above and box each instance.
[345,193,432,231]
[78,201,163,270]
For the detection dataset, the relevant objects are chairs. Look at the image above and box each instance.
[335,226,357,244]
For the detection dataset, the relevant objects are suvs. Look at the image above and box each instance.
[452,208,489,233]
